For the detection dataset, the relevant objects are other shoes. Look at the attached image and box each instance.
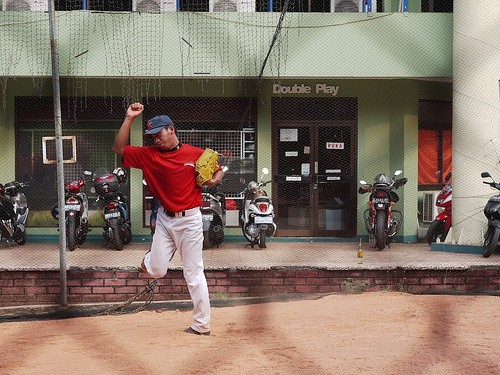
[184,327,210,335]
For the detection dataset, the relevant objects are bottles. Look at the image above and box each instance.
[357,242,363,264]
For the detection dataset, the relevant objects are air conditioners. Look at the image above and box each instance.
[132,0,177,12]
[330,0,377,13]
[422,192,444,222]
[208,0,255,12]
[2,0,49,11]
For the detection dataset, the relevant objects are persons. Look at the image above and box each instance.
[114,102,226,337]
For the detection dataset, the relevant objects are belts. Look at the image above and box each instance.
[163,209,184,218]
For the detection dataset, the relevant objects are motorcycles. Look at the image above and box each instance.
[200,165,230,247]
[237,167,278,249]
[83,171,131,251]
[51,178,91,252]
[0,180,32,247]
[356,169,409,251]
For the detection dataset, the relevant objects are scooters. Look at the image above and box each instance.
[479,172,500,256]
[141,178,156,236]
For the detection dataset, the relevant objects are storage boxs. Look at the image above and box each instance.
[92,174,118,195]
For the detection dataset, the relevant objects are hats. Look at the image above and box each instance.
[144,114,174,134]
